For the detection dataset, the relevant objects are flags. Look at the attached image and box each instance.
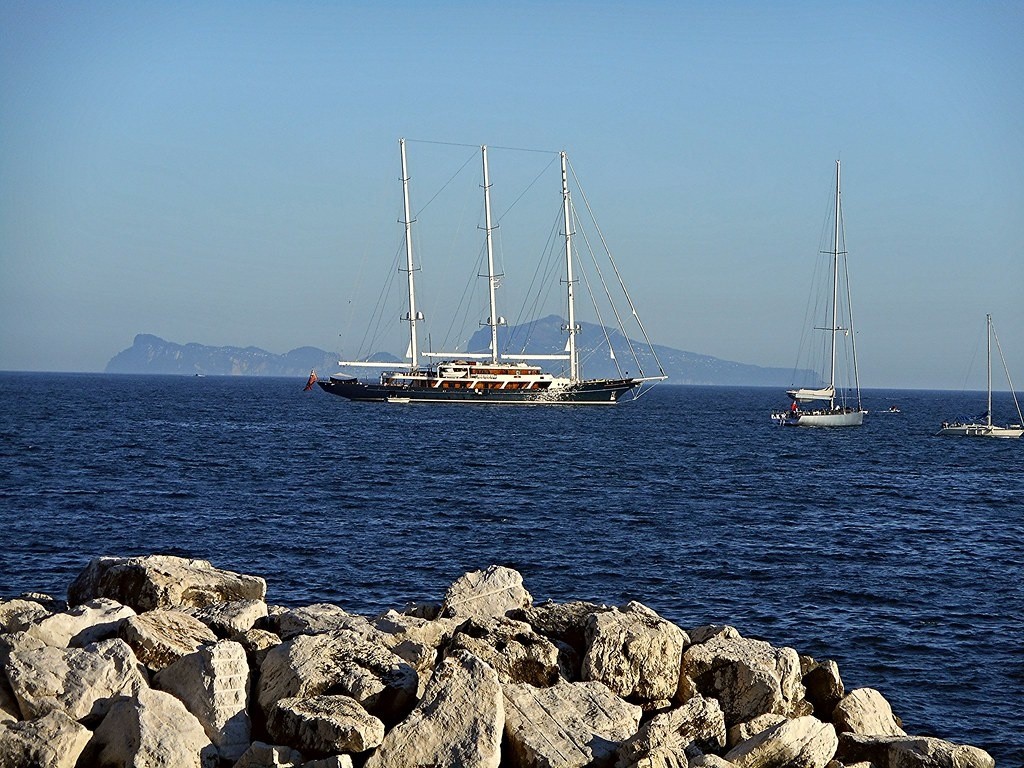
[303,369,317,391]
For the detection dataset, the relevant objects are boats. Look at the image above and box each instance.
[875,405,900,412]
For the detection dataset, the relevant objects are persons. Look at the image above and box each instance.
[888,405,899,411]
[942,420,961,428]
[427,368,433,388]
[1005,423,1010,430]
[772,401,864,426]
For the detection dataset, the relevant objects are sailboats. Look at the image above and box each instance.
[315,140,670,403]
[770,159,868,427]
[936,313,1024,438]
